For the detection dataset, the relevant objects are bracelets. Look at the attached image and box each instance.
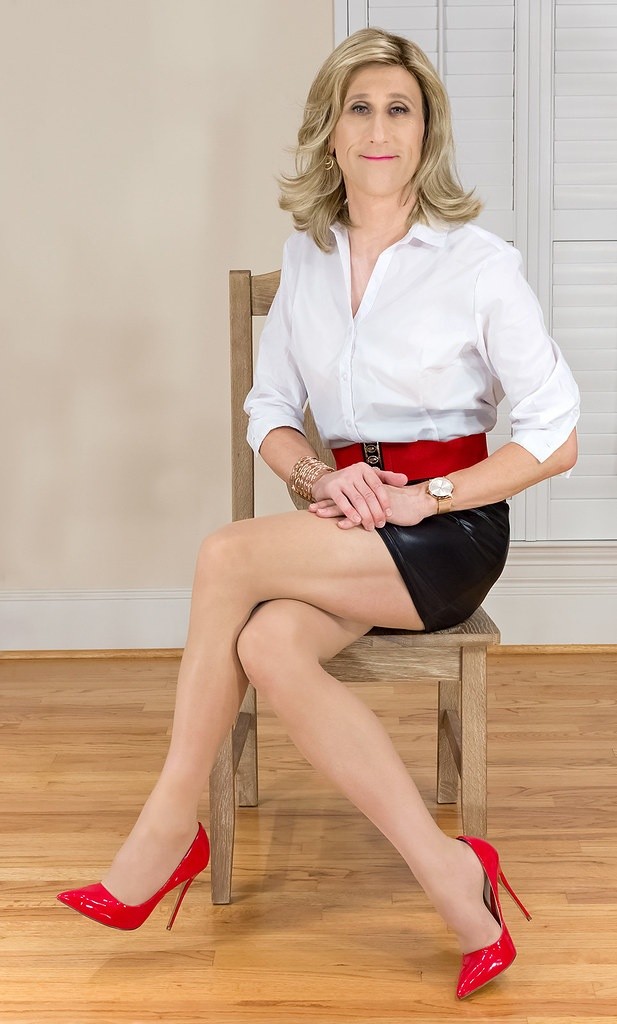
[291,455,335,502]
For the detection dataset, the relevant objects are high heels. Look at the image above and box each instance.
[450,836,533,999]
[55,822,211,931]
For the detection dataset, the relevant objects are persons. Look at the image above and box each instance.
[56,29,580,999]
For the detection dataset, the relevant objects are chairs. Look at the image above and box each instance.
[208,264,500,906]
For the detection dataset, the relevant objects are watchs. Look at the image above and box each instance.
[427,476,455,514]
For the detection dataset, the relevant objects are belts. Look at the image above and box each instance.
[324,434,488,485]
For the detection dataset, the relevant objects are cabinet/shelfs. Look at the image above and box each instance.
[329,2,614,645]
